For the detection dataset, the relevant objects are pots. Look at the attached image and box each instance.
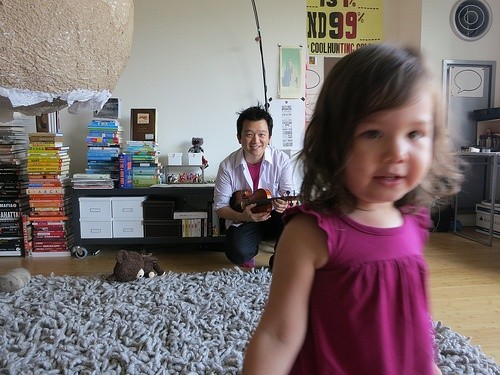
[479,128,500,152]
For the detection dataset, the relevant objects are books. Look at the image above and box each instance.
[70,118,164,189]
[0,121,77,252]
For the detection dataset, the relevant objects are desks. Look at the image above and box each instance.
[451,152,500,247]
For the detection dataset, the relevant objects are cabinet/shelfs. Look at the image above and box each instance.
[70,185,226,260]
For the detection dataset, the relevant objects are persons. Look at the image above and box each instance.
[211,107,297,270]
[242,44,442,375]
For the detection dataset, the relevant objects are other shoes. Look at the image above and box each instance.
[238,255,256,270]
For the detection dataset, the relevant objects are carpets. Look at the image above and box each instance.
[0,265,500,375]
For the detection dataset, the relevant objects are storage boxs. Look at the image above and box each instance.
[165,153,204,185]
[78,197,180,238]
[475,203,500,239]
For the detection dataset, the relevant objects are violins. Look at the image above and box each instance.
[230,188,306,213]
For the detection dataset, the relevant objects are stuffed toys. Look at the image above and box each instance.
[106,250,164,283]
[188,137,204,153]
